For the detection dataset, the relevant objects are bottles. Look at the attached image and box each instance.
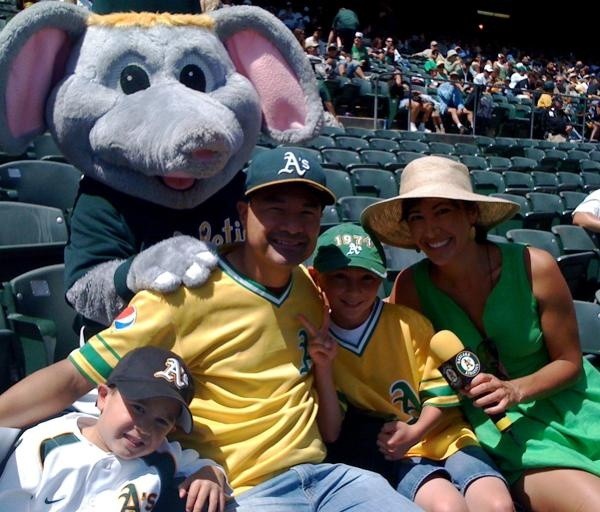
[384,116,388,129]
[418,122,425,131]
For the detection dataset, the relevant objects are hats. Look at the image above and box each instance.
[314,223,389,280]
[108,347,194,436]
[243,146,336,206]
[361,155,522,249]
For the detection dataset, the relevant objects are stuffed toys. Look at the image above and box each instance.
[1,0,325,347]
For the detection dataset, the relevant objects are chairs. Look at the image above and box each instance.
[0,124,599,384]
[314,35,599,121]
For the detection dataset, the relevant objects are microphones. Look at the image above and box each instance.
[429,330,513,433]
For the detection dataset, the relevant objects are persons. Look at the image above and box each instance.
[279,3,599,145]
[362,155,600,511]
[1,147,422,511]
[297,222,514,510]
[572,186,599,230]
[2,347,233,512]
